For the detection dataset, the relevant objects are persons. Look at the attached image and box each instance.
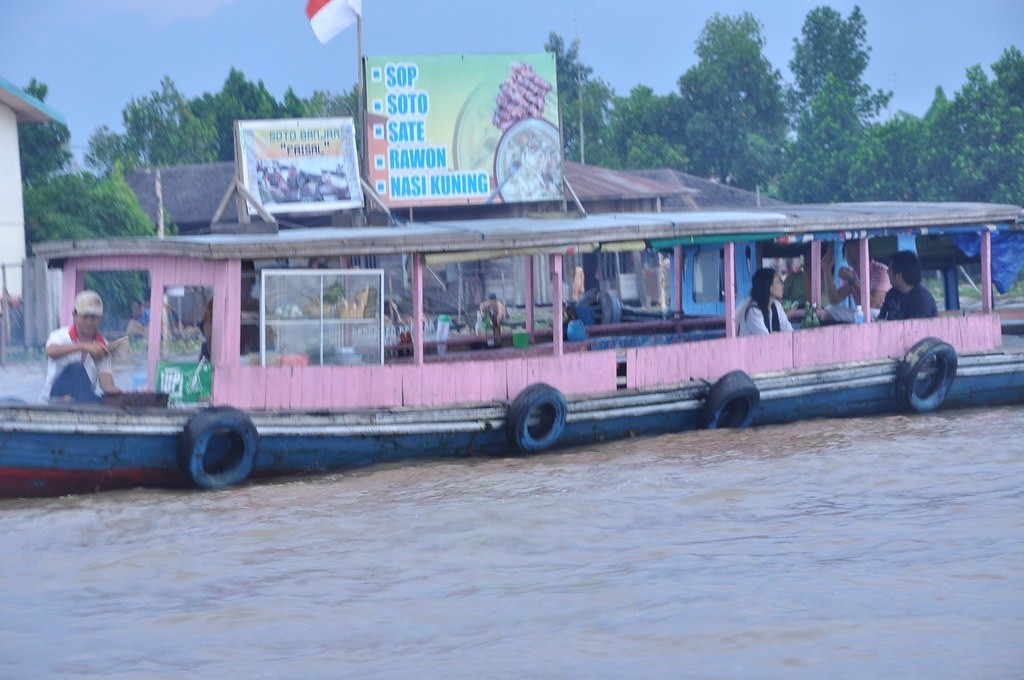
[37,291,121,402]
[257,160,351,204]
[821,239,893,325]
[735,267,793,336]
[480,293,506,328]
[126,303,179,336]
[199,259,275,363]
[876,250,938,321]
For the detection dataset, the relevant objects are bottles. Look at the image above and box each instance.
[800,302,813,328]
[485,319,495,349]
[436,315,450,342]
[386,319,436,345]
[475,310,484,337]
[855,305,864,323]
[811,303,820,327]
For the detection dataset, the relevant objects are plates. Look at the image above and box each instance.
[494,117,564,203]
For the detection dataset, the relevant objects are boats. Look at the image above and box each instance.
[0,200,1024,493]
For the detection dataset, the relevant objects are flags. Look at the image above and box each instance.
[305,0,361,45]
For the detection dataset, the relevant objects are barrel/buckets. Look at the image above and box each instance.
[512,333,529,349]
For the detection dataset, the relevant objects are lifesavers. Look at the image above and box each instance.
[176,404,261,491]
[505,381,568,455]
[696,368,761,430]
[894,336,958,415]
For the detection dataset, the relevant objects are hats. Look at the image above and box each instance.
[75,289,104,317]
